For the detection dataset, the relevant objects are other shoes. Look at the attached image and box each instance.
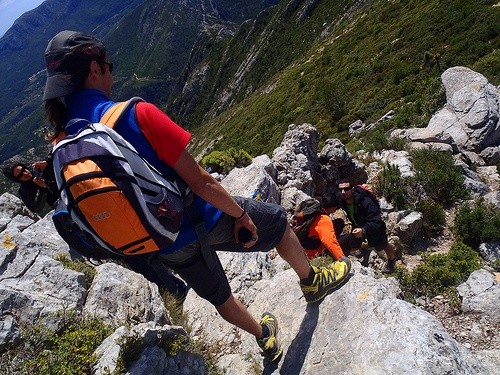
[384,259,396,274]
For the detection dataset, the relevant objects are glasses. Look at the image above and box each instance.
[338,187,352,192]
[102,60,114,73]
[17,166,26,180]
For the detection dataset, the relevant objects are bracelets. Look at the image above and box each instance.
[235,208,246,219]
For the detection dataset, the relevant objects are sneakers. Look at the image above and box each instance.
[299,256,351,305]
[255,312,282,361]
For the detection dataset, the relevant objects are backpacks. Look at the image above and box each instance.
[52,97,183,258]
[290,198,321,242]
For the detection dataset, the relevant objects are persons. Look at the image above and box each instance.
[40,123,190,296]
[42,29,352,362]
[290,192,353,261]
[2,152,63,215]
[337,179,397,273]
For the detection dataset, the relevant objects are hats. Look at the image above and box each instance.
[40,30,103,101]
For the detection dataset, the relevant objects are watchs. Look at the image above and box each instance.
[362,228,366,235]
